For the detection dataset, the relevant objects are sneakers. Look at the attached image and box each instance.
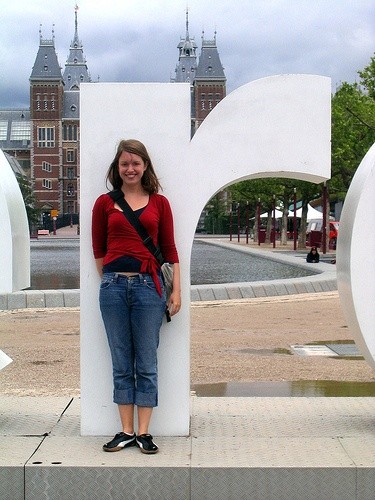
[136,433,159,454]
[103,432,136,452]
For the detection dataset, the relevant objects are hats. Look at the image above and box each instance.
[311,247,317,251]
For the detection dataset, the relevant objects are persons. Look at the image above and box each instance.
[92,140,181,454]
[306,247,320,263]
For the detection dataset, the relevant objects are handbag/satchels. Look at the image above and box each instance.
[160,263,174,291]
